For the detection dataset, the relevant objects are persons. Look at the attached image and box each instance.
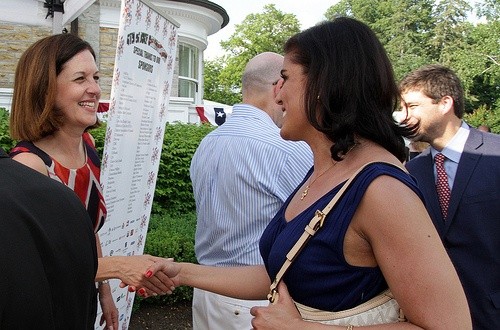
[0,147,98,330]
[8,33,176,329]
[119,15,472,330]
[189,52,314,330]
[398,64,500,330]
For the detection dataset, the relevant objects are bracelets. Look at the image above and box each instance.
[99,279,109,285]
[346,323,353,330]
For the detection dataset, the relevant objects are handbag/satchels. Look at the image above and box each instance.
[268,160,412,326]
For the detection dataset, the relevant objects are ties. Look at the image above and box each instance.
[435,153,451,221]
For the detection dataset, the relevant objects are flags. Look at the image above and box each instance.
[195,106,234,126]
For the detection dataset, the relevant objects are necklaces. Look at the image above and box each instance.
[300,143,357,200]
